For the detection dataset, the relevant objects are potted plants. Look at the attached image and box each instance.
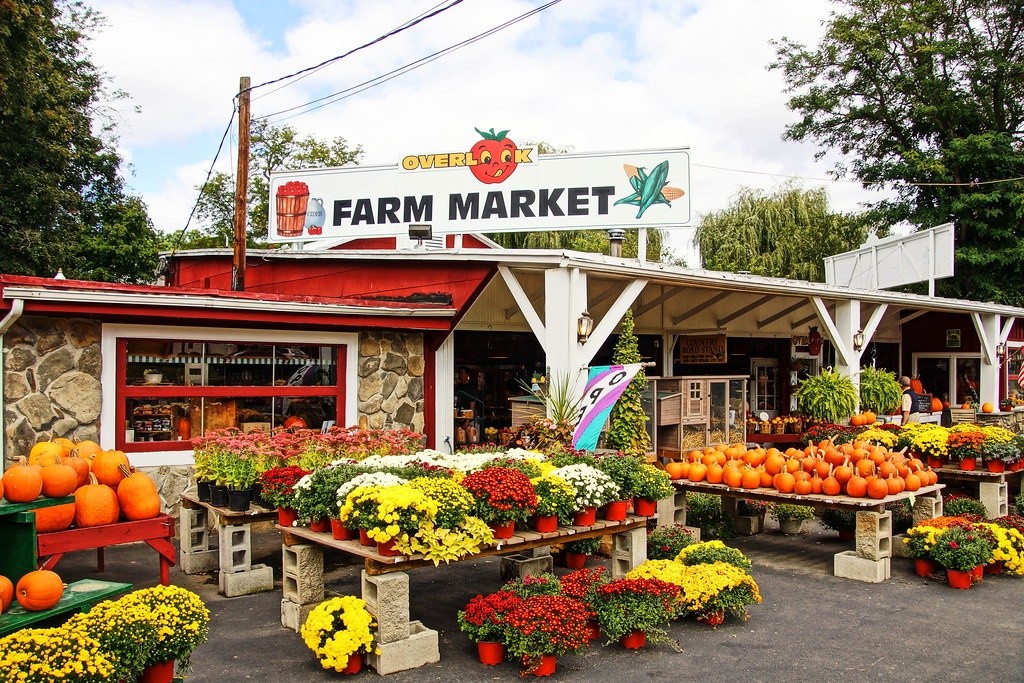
[605,307,652,453]
[768,502,814,535]
[792,364,905,425]
[999,398,1016,417]
[143,369,163,383]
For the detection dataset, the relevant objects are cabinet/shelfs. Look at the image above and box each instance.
[131,412,178,441]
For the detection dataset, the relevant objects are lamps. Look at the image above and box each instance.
[578,309,593,343]
[997,342,1005,358]
[854,330,864,352]
[654,336,662,351]
[488,333,509,359]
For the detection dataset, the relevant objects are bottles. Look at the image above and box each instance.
[178,411,190,440]
[455,425,478,443]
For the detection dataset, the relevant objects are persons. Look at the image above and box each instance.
[899,376,919,426]
[455,367,480,442]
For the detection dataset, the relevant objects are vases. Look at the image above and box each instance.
[735,515,764,535]
[915,559,1003,589]
[907,450,1024,472]
[144,661,174,683]
[197,477,727,677]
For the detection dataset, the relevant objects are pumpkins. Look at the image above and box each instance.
[961,402,970,409]
[284,415,307,428]
[931,397,943,411]
[982,403,993,413]
[0,575,14,616]
[850,409,876,426]
[178,416,190,440]
[910,376,922,394]
[16,570,69,611]
[0,435,162,534]
[665,433,938,501]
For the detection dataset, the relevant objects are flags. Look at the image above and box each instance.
[573,365,640,451]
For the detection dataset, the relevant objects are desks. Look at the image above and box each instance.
[976,412,1014,428]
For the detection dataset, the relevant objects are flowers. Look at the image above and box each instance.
[816,494,1024,576]
[0,369,776,679]
[800,422,1024,455]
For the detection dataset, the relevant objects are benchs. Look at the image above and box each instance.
[949,408,977,427]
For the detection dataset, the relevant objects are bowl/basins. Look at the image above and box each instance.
[147,373,162,383]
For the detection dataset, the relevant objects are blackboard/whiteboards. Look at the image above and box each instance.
[915,393,933,412]
